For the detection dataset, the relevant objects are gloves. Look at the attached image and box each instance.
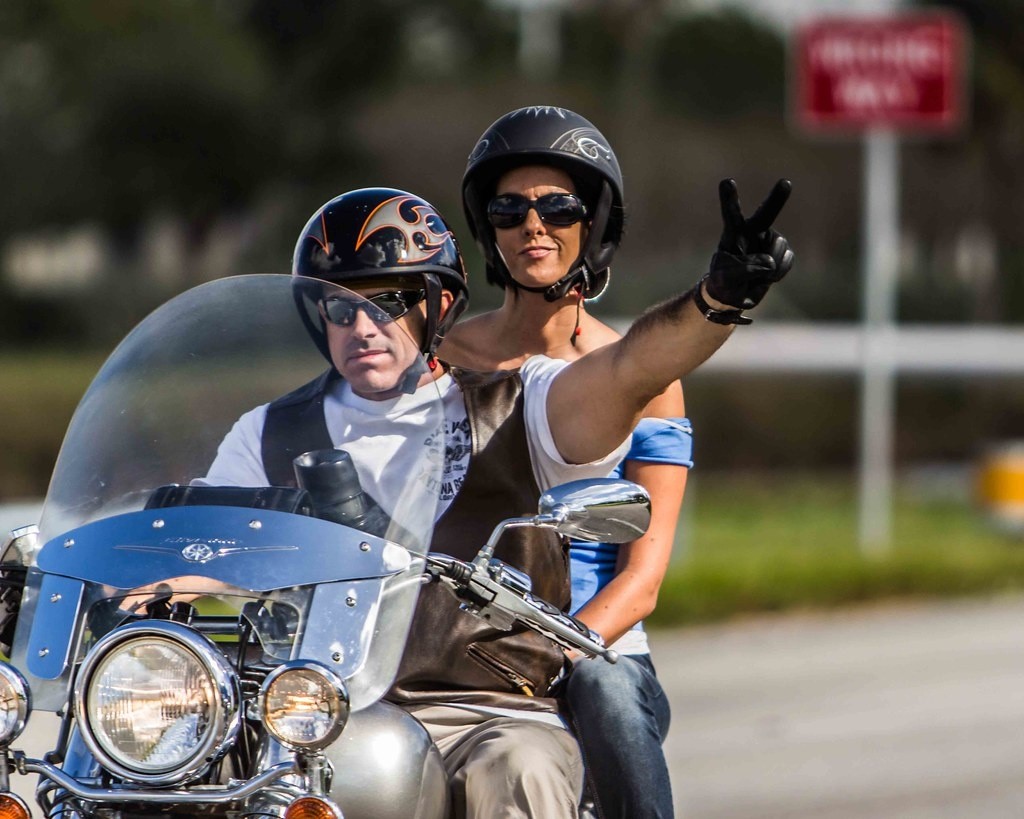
[705,176,797,312]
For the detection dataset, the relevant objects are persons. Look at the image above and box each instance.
[189,179,796,819]
[433,105,695,819]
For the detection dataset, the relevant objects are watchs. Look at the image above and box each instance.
[694,274,753,327]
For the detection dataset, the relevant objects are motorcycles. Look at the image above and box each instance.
[0,274,652,817]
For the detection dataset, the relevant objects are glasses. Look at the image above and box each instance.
[320,289,426,328]
[487,192,589,230]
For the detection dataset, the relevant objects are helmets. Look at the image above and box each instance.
[290,185,471,396]
[460,105,626,347]
